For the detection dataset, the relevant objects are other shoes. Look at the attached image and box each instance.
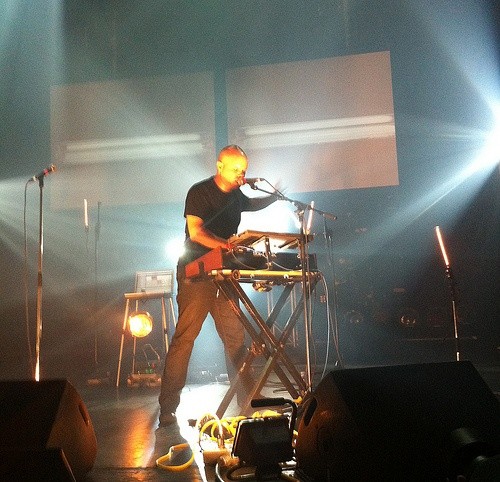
[159,412,176,425]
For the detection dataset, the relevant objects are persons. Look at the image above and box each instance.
[157,144,289,428]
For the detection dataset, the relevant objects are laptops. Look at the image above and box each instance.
[134,270,174,292]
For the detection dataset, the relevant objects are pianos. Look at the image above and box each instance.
[184,246,320,421]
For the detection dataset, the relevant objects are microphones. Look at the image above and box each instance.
[29,164,57,182]
[307,201,315,234]
[434,225,450,268]
[83,198,88,232]
[238,178,265,185]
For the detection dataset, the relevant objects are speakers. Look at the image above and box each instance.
[0,379,97,482]
[301,361,500,482]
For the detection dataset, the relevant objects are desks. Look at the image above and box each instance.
[215,270,322,420]
[117,291,178,387]
